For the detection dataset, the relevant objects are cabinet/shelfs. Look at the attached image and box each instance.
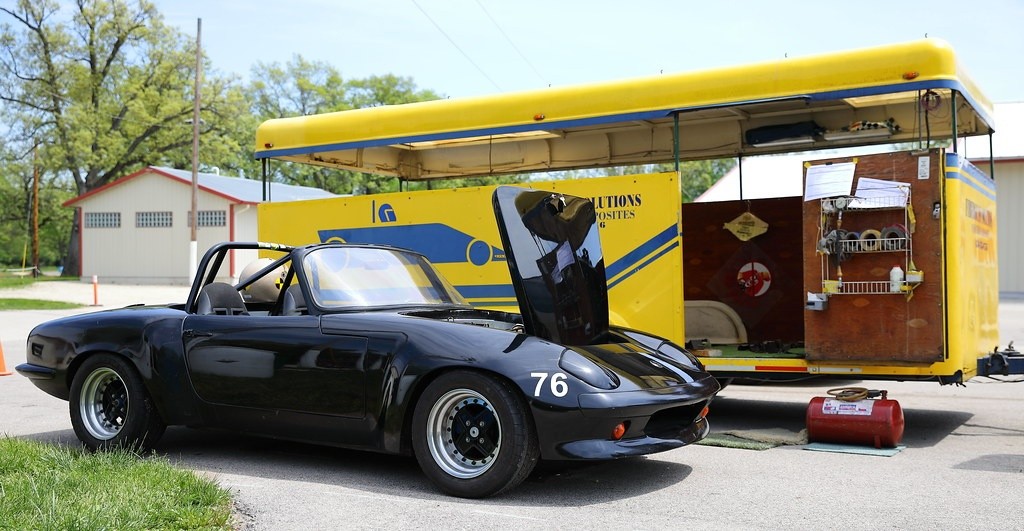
[820,188,912,294]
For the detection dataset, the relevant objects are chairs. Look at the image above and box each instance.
[285,285,322,317]
[193,282,249,316]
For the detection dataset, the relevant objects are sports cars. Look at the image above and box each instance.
[14,184,724,500]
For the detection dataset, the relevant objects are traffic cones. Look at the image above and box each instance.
[0,341,14,376]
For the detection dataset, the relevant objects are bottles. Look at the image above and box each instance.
[889,265,904,292]
